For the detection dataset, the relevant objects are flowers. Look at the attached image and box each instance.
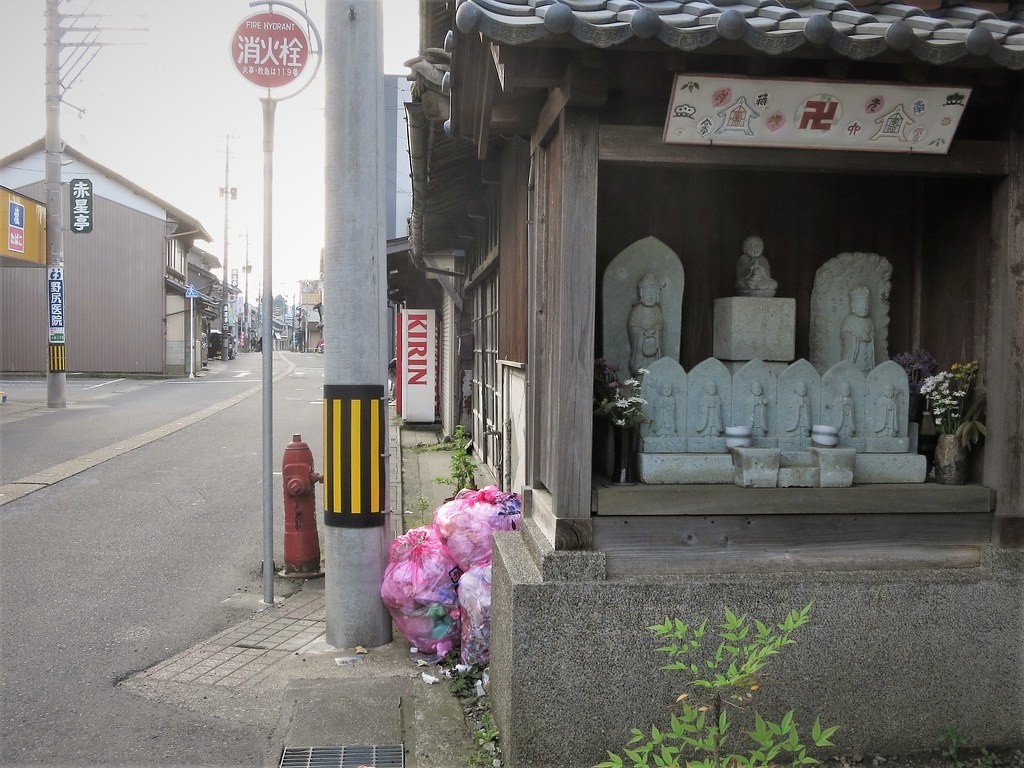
[591,358,650,428]
[918,359,987,450]
[893,348,938,395]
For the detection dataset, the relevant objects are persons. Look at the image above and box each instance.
[832,383,856,438]
[628,273,664,377]
[786,381,811,438]
[730,236,778,290]
[841,286,875,376]
[875,384,900,438]
[696,381,724,439]
[653,383,677,438]
[747,381,768,438]
[388,351,396,403]
[251,337,256,351]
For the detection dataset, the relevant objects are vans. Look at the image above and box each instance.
[208,332,238,359]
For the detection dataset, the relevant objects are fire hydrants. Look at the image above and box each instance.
[277,435,325,580]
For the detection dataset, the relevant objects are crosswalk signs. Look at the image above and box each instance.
[186,284,198,297]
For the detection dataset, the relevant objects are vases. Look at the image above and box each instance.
[609,425,638,486]
[936,433,968,486]
[910,394,919,422]
